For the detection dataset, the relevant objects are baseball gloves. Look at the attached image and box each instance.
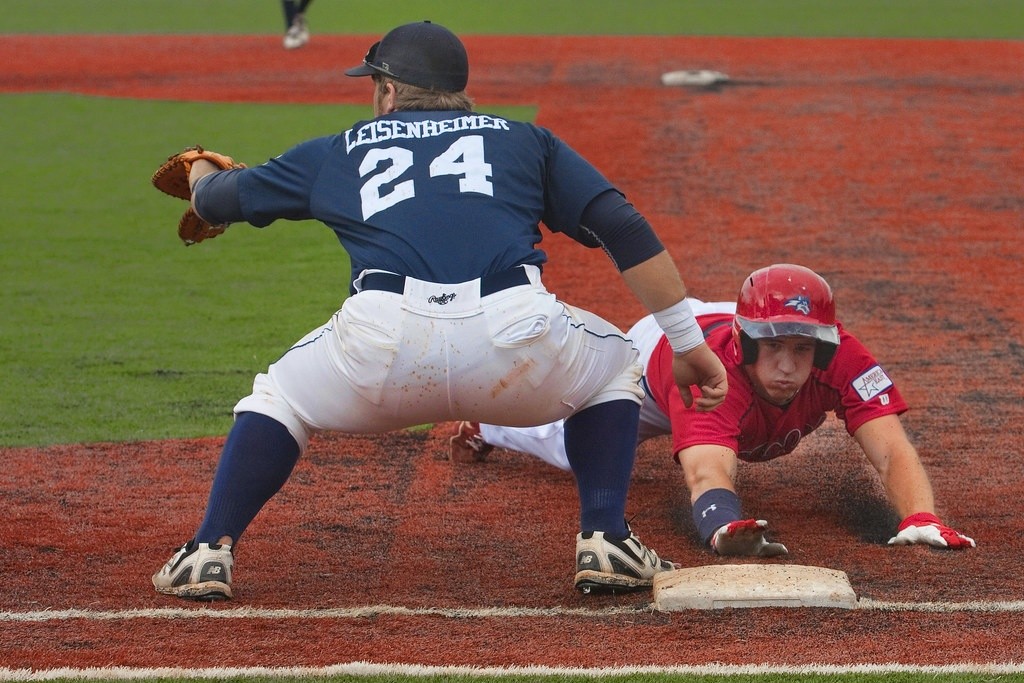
[148,145,249,247]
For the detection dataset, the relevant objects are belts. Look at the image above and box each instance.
[361,267,531,300]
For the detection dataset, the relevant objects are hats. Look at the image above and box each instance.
[343,20,469,92]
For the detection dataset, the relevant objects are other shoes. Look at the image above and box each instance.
[283,13,310,48]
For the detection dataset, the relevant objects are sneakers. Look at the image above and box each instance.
[449,421,495,466]
[151,540,234,601]
[573,514,681,598]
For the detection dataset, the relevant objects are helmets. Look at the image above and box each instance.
[732,263,840,371]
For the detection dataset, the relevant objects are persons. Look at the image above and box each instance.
[448,263,978,557]
[151,20,728,600]
[280,0,315,50]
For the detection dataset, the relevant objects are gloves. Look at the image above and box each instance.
[888,512,976,550]
[710,519,788,559]
